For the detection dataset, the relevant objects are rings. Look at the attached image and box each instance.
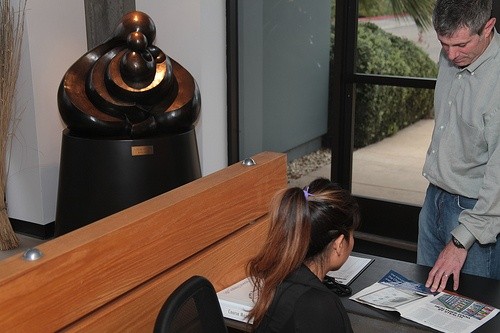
[443,274,450,277]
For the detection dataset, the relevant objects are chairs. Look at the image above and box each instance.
[152,275,228,333]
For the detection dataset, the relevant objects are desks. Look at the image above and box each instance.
[220,248,500,333]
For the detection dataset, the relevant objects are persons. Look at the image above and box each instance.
[243,178,360,333]
[416,0,500,292]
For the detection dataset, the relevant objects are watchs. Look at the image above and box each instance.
[452,236,465,248]
